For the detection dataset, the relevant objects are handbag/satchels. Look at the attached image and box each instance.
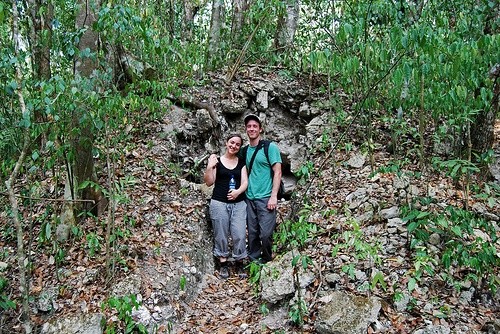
[277,179,285,200]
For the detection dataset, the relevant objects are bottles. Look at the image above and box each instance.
[229,175,236,193]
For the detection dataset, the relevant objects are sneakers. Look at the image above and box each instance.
[219,264,245,277]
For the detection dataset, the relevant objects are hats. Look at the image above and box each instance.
[244,114,262,127]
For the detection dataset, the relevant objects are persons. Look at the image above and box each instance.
[204,134,248,279]
[209,114,282,277]
